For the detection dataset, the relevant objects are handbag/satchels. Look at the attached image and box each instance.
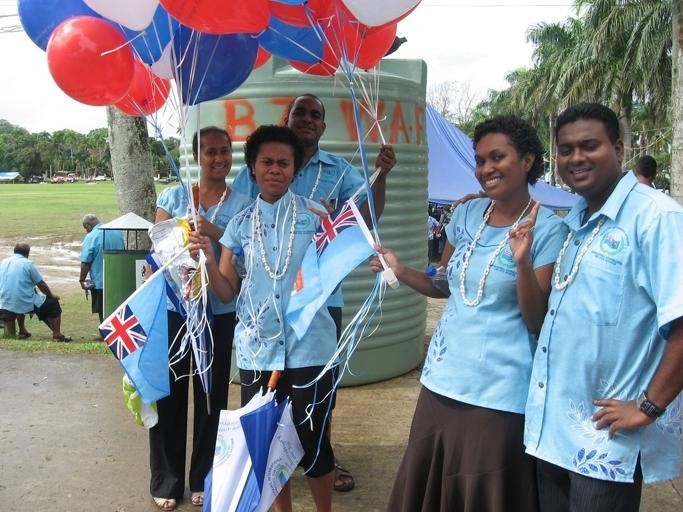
[121,371,159,430]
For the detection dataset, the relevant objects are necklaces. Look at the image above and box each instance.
[308,160,323,198]
[555,215,605,289]
[459,195,532,306]
[192,180,229,228]
[254,189,296,278]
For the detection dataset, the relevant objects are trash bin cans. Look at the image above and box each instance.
[98,211,155,321]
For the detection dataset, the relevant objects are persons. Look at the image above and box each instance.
[80,214,126,342]
[637,154,657,189]
[456,103,683,512]
[233,93,397,493]
[369,117,568,510]
[1,242,72,342]
[144,127,254,510]
[427,202,455,260]
[188,124,340,511]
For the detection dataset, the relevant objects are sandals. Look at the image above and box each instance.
[190,491,203,505]
[152,495,176,510]
[333,457,354,491]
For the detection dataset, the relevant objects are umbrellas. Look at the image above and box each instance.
[202,371,305,509]
[146,248,216,414]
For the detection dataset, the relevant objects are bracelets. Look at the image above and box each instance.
[79,279,84,282]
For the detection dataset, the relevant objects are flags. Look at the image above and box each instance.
[286,200,379,342]
[97,271,172,405]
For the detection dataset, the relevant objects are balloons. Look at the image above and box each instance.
[18,0,423,119]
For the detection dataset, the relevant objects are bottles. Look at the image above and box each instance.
[425,266,450,297]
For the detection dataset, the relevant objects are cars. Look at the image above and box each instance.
[23,169,111,183]
[535,171,578,196]
[152,170,178,183]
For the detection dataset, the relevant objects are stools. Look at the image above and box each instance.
[0,308,36,339]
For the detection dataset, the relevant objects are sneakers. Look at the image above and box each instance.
[18,331,30,338]
[53,335,72,341]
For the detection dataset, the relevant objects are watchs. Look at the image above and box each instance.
[635,390,663,418]
